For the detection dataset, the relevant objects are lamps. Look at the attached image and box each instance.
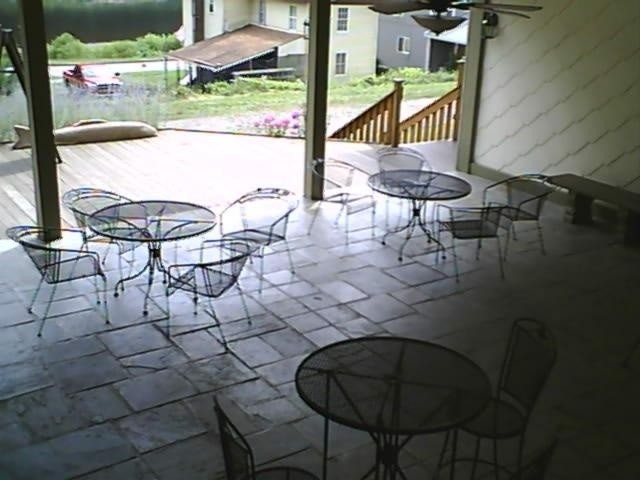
[481,9,500,40]
[411,7,469,37]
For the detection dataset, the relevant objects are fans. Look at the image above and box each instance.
[327,1,545,21]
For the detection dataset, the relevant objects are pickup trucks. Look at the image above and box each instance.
[63,62,125,100]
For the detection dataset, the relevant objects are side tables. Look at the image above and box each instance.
[89,200,218,319]
[366,169,473,264]
[296,334,494,478]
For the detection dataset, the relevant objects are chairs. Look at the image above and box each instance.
[5,225,111,337]
[377,148,434,227]
[61,188,148,293]
[210,396,319,479]
[220,187,300,292]
[435,202,514,285]
[436,316,561,478]
[165,238,263,353]
[477,173,557,252]
[307,159,376,245]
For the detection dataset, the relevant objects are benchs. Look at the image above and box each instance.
[548,168,639,245]
